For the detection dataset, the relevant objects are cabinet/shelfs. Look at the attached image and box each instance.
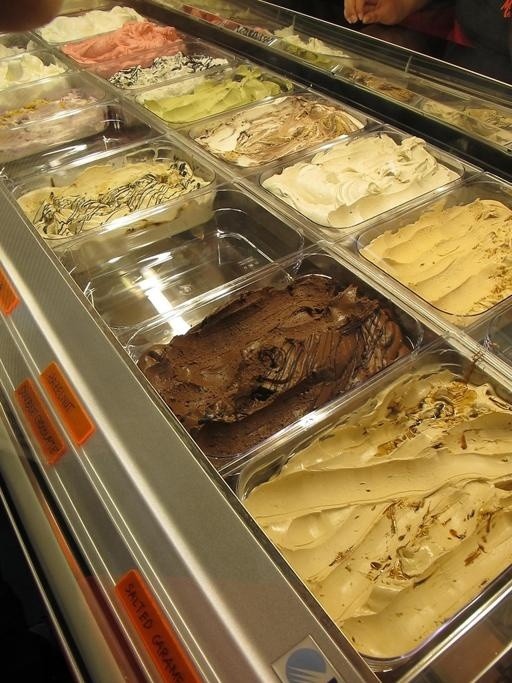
[0,0,512,678]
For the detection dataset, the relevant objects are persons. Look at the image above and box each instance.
[0,0,65,34]
[344,0,512,55]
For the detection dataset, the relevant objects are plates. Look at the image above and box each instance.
[131,58,285,128]
[11,139,216,242]
[1,73,107,129]
[91,39,218,91]
[1,50,71,88]
[60,23,184,65]
[60,189,307,331]
[232,350,510,673]
[256,128,467,231]
[0,103,155,184]
[353,182,512,318]
[27,4,148,47]
[485,304,512,369]
[124,251,425,460]
[185,91,370,170]
